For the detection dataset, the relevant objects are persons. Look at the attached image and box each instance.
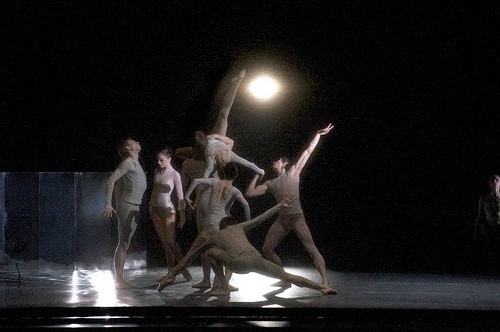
[100,138,147,289]
[473,174,500,271]
[151,68,338,296]
